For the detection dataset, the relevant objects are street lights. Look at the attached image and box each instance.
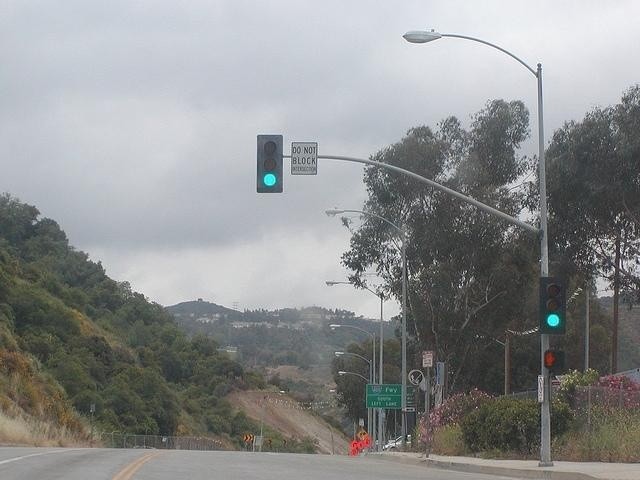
[403,28,558,469]
[321,206,411,451]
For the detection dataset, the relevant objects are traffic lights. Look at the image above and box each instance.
[542,349,565,372]
[536,272,567,339]
[255,134,285,194]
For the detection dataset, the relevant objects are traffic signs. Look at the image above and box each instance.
[290,141,318,177]
